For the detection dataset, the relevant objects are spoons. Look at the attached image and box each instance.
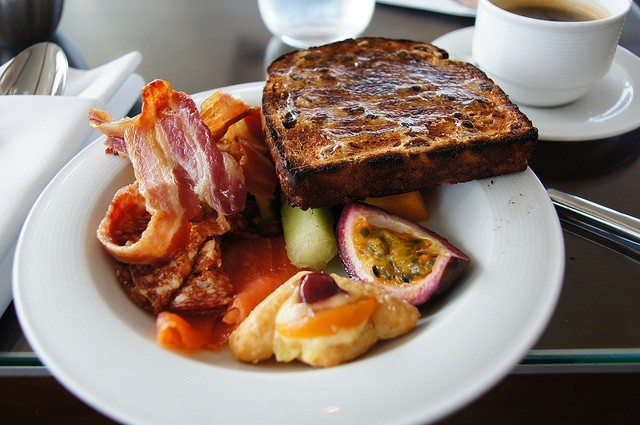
[0,39,69,96]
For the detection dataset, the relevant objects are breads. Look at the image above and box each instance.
[262,37,538,211]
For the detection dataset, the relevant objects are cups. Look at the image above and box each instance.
[471,0,633,109]
[257,0,377,50]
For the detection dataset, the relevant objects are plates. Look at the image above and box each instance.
[431,24,640,143]
[10,80,568,425]
